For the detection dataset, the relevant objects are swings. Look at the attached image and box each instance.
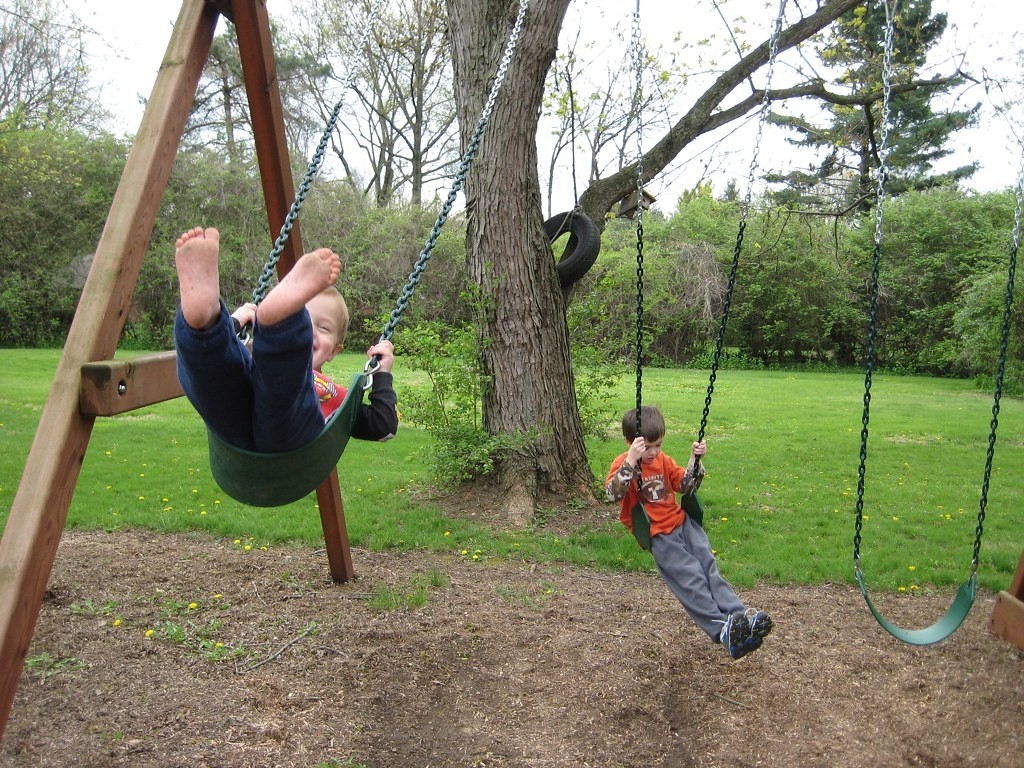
[849,0,1024,650]
[628,0,790,548]
[196,0,529,509]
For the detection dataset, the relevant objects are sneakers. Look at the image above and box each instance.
[745,608,772,652]
[712,612,753,660]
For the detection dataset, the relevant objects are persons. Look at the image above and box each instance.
[174,226,397,508]
[605,406,772,659]
